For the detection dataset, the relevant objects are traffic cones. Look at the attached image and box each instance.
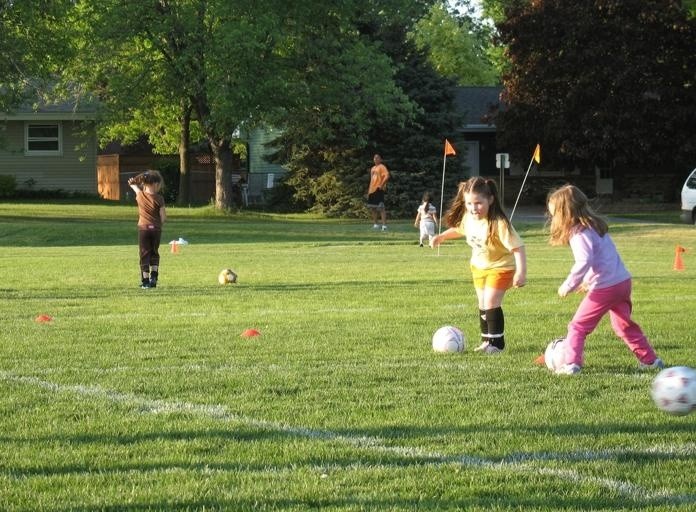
[672,246,686,270]
[167,239,180,254]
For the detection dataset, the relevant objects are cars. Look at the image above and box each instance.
[680,167,696,225]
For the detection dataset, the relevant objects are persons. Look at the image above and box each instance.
[430,178,526,353]
[127,171,166,289]
[367,153,390,231]
[547,185,664,376]
[414,192,437,247]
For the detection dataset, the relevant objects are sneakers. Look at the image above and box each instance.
[486,346,503,354]
[474,340,489,351]
[640,358,664,369]
[141,284,150,289]
[150,277,156,287]
[568,364,580,375]
[373,225,379,230]
[381,226,387,230]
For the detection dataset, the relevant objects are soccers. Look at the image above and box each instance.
[433,326,465,352]
[651,366,696,412]
[545,339,568,370]
